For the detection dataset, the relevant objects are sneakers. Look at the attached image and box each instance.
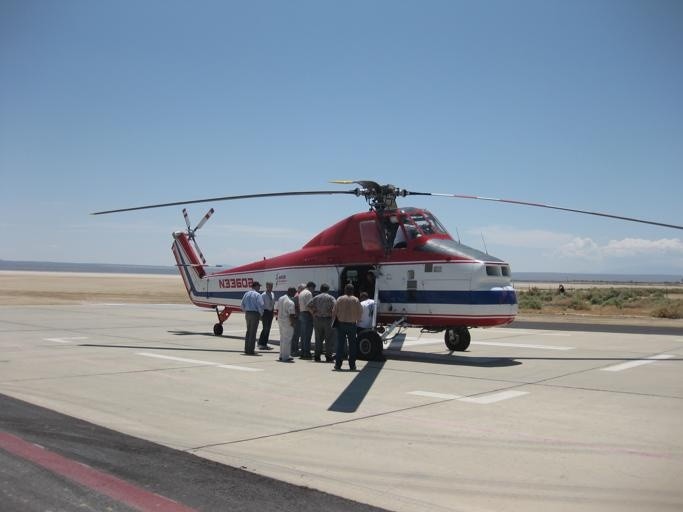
[331,366,339,371]
[279,357,293,360]
[350,367,356,372]
[314,354,335,361]
[260,347,273,350]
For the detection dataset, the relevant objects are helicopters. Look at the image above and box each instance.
[89,179,682,351]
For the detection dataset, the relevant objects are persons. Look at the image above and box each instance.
[275,282,375,370]
[257,282,275,349]
[393,216,431,247]
[240,281,264,353]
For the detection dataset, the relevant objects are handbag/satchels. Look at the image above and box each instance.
[331,316,337,328]
